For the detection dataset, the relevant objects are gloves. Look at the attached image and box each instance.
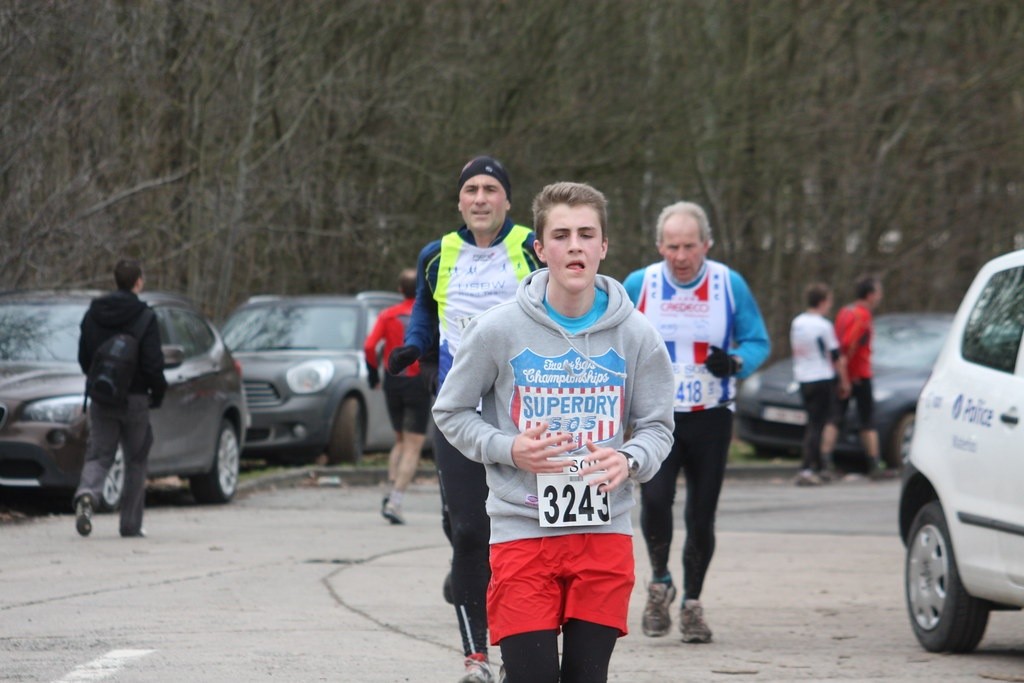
[388,345,421,375]
[704,346,738,379]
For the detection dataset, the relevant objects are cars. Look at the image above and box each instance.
[734,309,957,472]
[215,289,436,468]
[896,249,1024,658]
[1,286,249,516]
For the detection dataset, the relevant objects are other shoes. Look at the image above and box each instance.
[76,495,93,536]
[796,470,821,485]
[382,498,404,524]
[870,462,900,479]
[120,530,145,537]
[821,469,839,480]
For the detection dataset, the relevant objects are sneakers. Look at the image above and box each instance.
[498,665,507,683]
[642,584,676,637]
[678,599,712,642]
[458,653,495,683]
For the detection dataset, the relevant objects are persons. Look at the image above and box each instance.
[387,154,545,683]
[365,268,429,525]
[789,281,851,487]
[432,184,676,683]
[820,279,902,483]
[621,200,771,640]
[72,260,166,537]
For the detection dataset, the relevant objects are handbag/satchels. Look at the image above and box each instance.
[85,334,140,407]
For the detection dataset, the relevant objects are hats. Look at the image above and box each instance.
[457,156,511,202]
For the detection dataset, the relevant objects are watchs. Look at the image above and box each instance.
[619,451,638,475]
